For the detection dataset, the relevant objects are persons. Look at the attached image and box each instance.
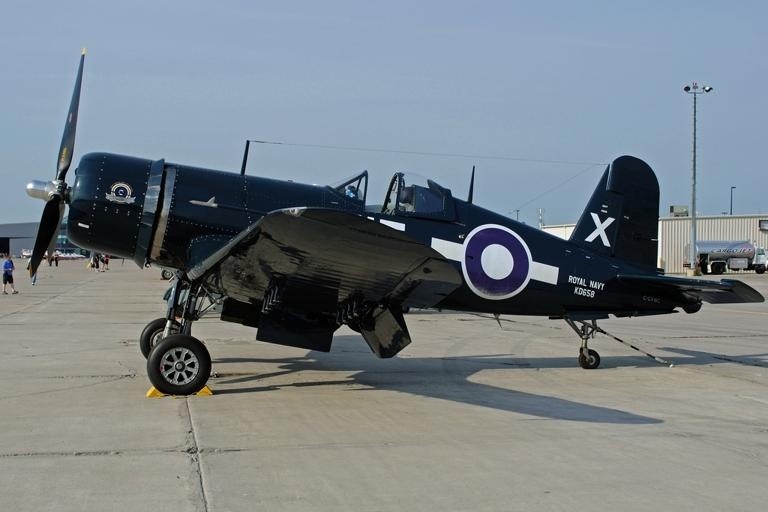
[85,253,110,272]
[54,249,59,267]
[3,256,19,294]
[26,257,36,285]
[48,257,54,266]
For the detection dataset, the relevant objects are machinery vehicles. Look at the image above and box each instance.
[680,238,767,275]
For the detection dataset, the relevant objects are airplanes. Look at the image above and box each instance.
[18,40,764,403]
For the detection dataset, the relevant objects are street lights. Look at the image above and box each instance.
[728,186,735,215]
[681,79,716,279]
